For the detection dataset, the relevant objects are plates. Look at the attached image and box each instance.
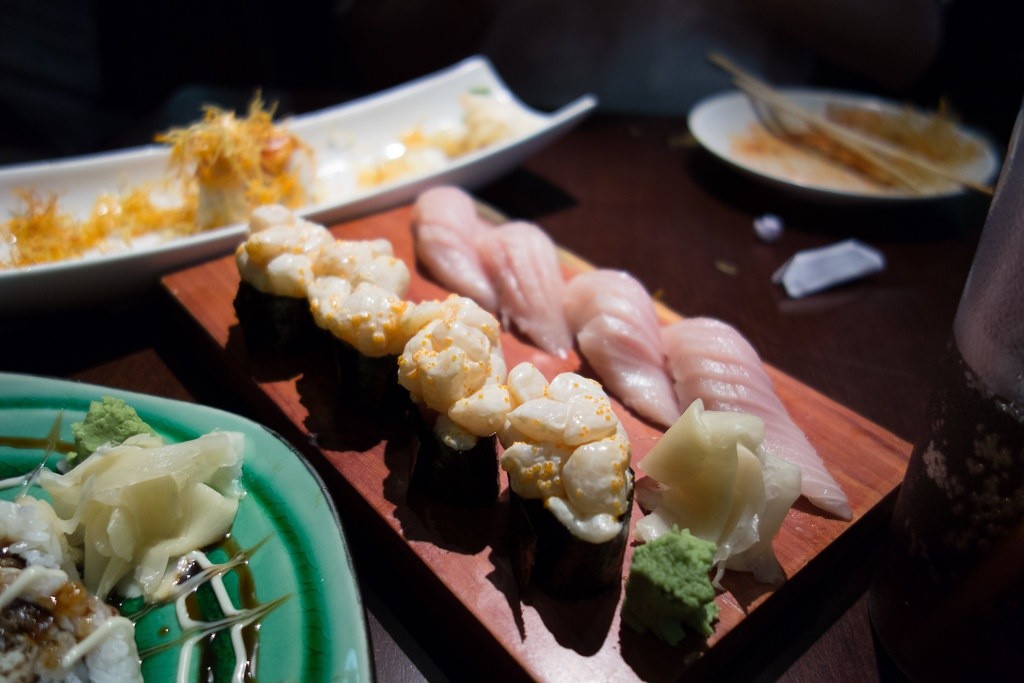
[0,367,377,682]
[0,54,598,313]
[160,185,912,682]
[689,83,1000,199]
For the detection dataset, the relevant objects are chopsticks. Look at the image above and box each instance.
[705,51,993,199]
